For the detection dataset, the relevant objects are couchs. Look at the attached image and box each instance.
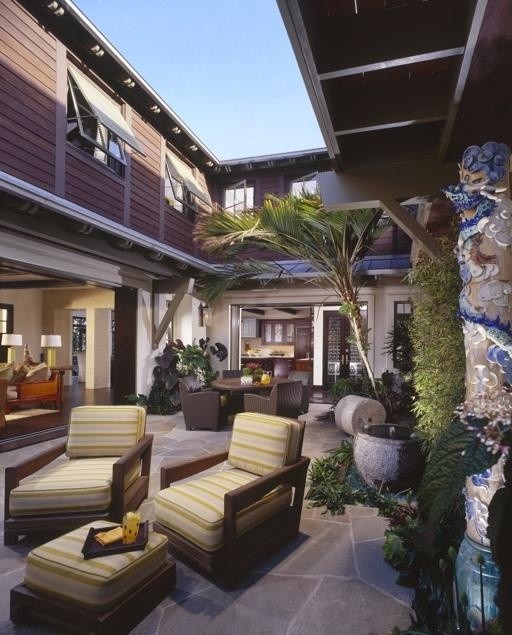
[2,401,154,547]
[151,410,312,581]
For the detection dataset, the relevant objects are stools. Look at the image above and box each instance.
[7,517,179,631]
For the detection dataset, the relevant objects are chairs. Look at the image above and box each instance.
[176,366,314,434]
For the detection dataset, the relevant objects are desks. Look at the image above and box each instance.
[241,356,294,380]
